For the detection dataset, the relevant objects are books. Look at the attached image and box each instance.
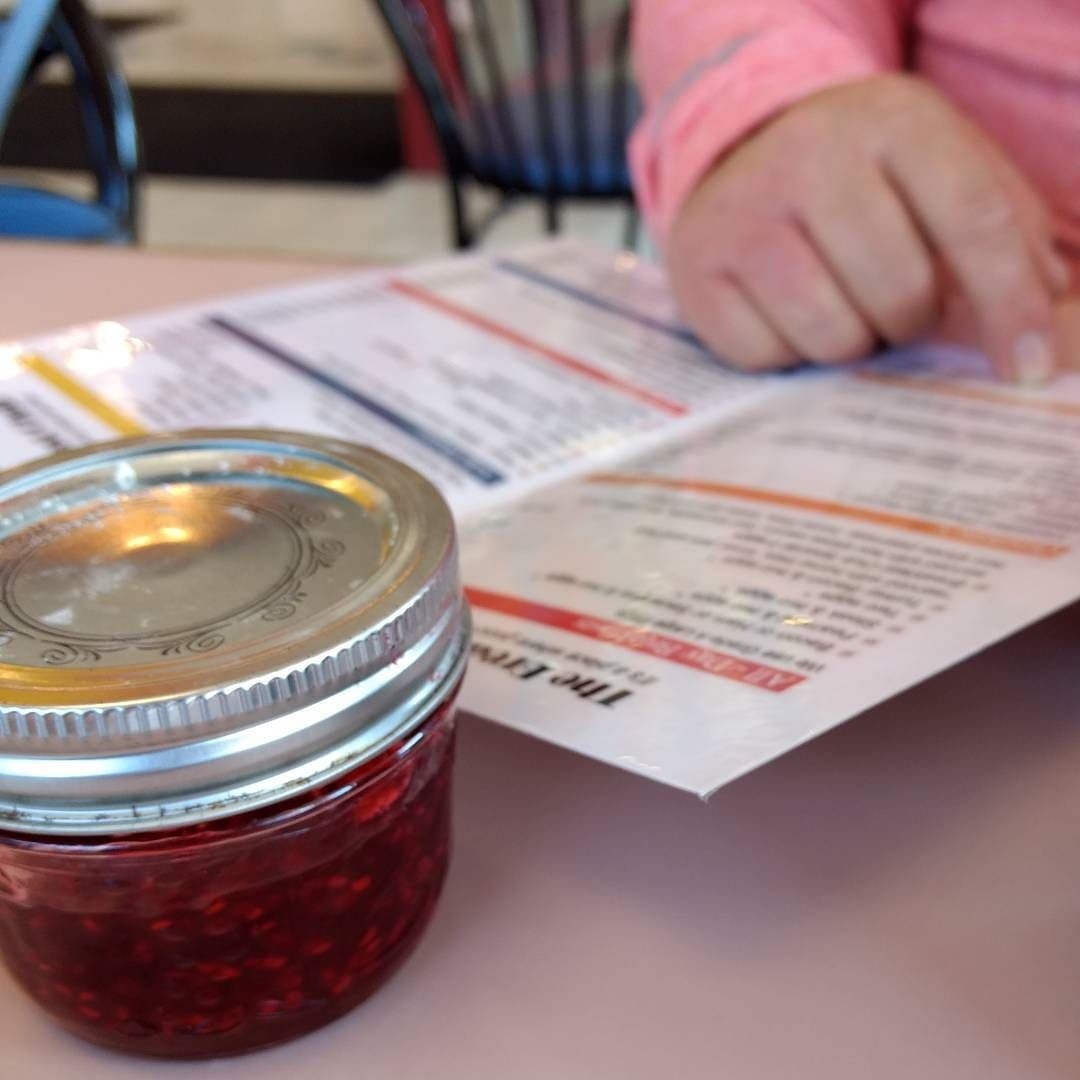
[0,244,1079,801]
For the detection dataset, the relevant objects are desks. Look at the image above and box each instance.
[0,234,1080,1080]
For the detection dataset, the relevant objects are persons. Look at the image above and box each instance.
[625,1,1080,382]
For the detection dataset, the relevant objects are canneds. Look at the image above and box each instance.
[1,429,470,1060]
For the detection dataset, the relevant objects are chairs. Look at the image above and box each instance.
[373,0,644,252]
